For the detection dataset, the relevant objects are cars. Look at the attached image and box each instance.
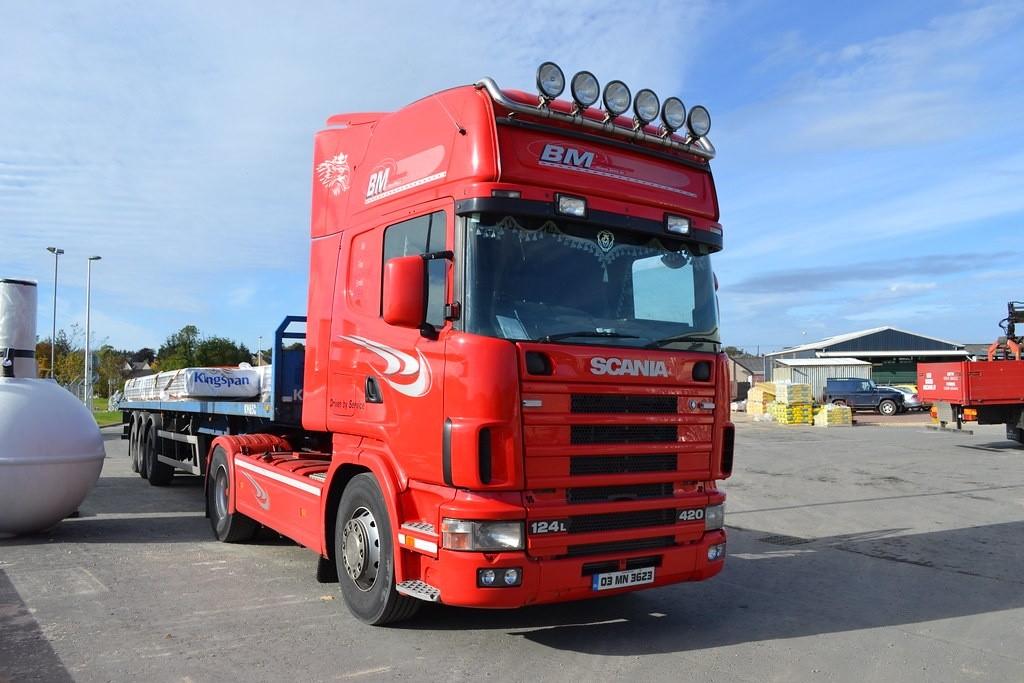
[876,384,932,412]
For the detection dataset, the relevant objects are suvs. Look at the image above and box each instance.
[822,377,904,415]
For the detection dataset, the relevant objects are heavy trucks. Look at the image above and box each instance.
[117,62,738,628]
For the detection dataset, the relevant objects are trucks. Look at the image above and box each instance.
[915,299,1024,443]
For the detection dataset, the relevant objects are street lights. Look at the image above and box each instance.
[45,246,66,378]
[84,255,102,405]
